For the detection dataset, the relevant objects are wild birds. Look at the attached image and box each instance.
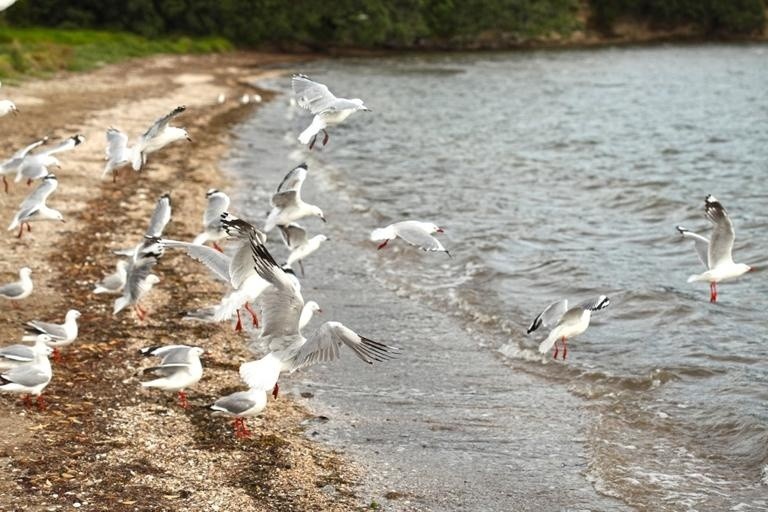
[675,194,756,303]
[103,104,193,178]
[1,97,81,399]
[368,220,452,256]
[286,69,370,153]
[95,164,388,433]
[526,293,611,360]
